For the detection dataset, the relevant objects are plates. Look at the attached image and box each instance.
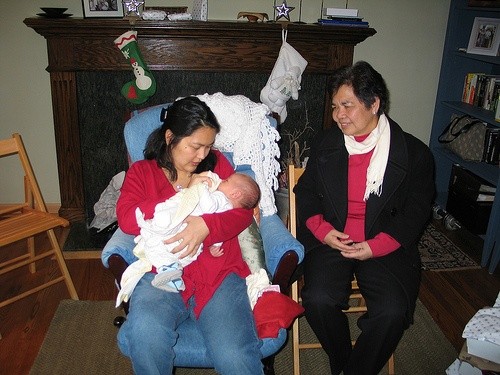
[36,13,74,17]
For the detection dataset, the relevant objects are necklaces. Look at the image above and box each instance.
[176,185,183,191]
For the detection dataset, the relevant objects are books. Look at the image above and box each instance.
[318,7,370,28]
[461,72,500,124]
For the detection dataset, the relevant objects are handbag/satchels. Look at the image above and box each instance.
[438,111,488,161]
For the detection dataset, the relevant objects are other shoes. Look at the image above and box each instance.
[153,262,183,286]
[151,277,183,293]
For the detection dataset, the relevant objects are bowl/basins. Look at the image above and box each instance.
[40,7,69,13]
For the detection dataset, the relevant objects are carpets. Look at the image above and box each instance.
[418,224,481,271]
[31,298,459,375]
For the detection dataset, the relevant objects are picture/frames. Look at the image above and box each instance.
[467,16,500,57]
[81,0,124,19]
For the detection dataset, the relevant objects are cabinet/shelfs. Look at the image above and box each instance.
[429,0,500,275]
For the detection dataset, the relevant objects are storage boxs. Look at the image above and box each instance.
[323,8,359,17]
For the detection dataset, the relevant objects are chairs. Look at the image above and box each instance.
[124,92,394,375]
[0,132,79,308]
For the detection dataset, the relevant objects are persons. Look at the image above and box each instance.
[476,28,496,49]
[139,172,262,292]
[116,98,266,375]
[292,61,437,375]
[101,0,109,10]
